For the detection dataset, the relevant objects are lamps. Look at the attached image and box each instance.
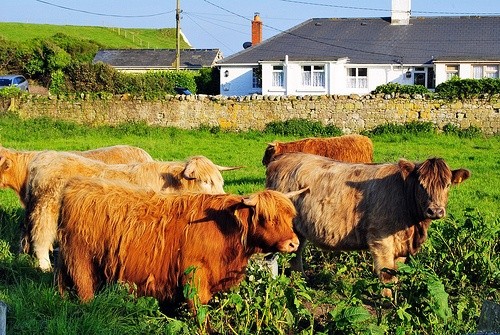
[225,70,229,77]
[406,68,412,78]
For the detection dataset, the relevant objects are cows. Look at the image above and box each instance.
[0,144,155,257]
[261,132,374,168]
[264,150,472,301]
[24,149,244,277]
[55,177,311,319]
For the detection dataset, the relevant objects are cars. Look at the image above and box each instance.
[174,87,193,95]
[0,74,29,93]
